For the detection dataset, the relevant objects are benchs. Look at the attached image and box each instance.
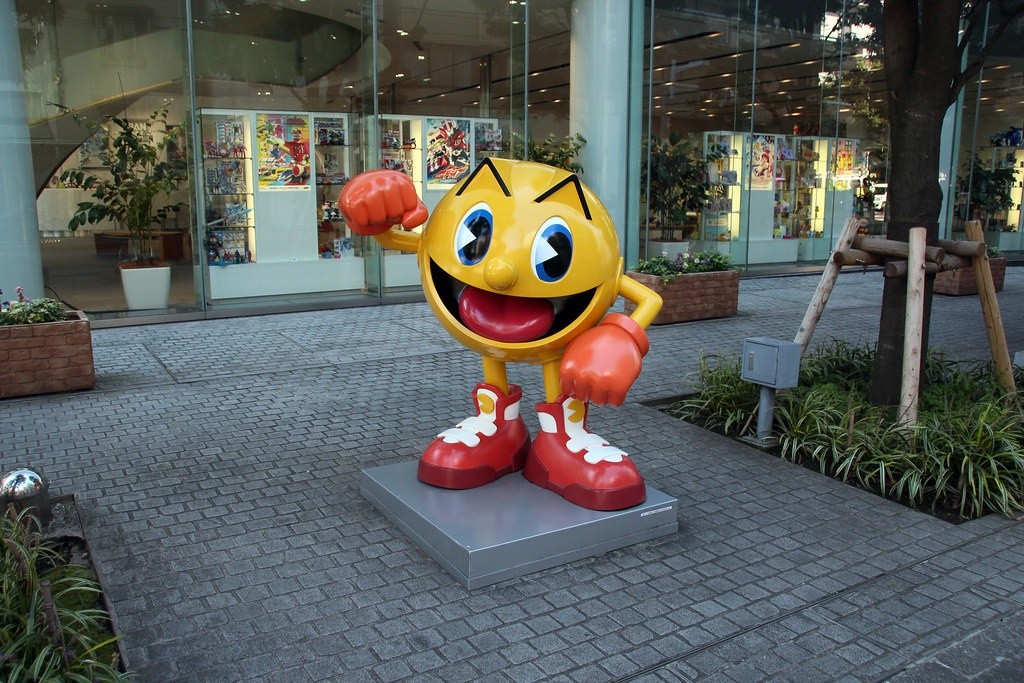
[91,229,181,265]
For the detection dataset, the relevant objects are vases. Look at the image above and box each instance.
[626,270,739,324]
[0,311,95,398]
[933,256,1008,296]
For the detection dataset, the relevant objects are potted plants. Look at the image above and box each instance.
[642,142,730,260]
[46,73,187,311]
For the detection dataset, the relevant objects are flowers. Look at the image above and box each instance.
[987,246,1001,258]
[628,247,732,286]
[0,287,69,324]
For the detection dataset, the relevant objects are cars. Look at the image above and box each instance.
[869,183,889,210]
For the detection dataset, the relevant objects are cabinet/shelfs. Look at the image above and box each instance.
[791,136,864,262]
[354,114,500,288]
[703,129,799,263]
[193,105,364,300]
[978,144,1024,251]
